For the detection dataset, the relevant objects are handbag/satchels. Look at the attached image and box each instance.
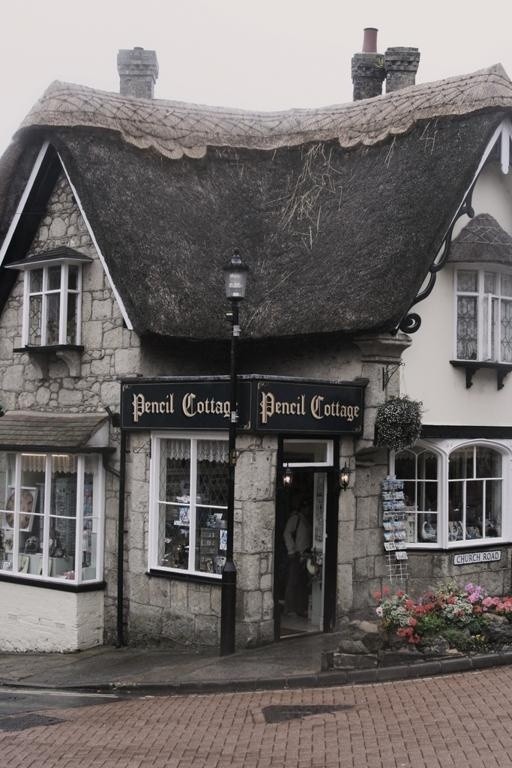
[275,520,288,570]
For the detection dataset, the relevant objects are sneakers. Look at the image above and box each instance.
[284,606,307,617]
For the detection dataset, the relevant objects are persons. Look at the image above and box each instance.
[281,496,312,619]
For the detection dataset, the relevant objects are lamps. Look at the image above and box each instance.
[277,457,294,489]
[329,462,351,492]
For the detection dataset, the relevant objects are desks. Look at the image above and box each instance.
[7,551,74,578]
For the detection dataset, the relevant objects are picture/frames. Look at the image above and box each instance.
[1,484,39,533]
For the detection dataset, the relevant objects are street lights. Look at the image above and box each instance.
[221,243,253,657]
